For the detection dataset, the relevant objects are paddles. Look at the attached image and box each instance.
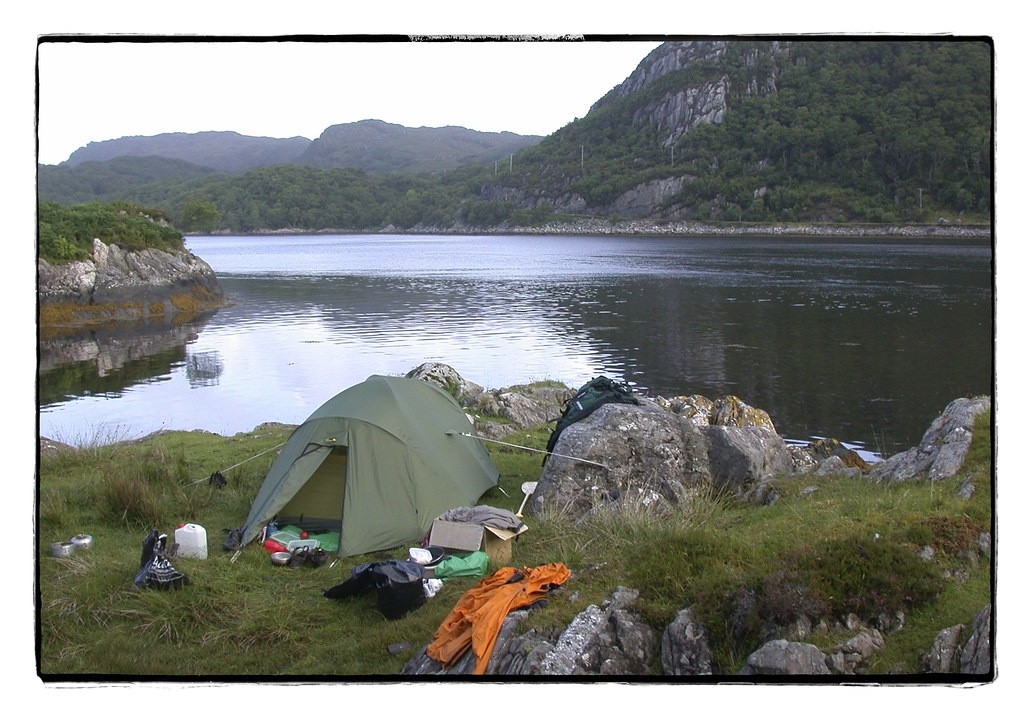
[515,481,538,518]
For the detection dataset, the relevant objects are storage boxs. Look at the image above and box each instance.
[427,512,529,568]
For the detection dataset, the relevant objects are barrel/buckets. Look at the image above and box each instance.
[174,523,208,560]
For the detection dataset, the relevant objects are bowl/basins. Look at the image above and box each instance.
[71,534,95,550]
[271,552,292,565]
[423,546,444,566]
[51,541,74,557]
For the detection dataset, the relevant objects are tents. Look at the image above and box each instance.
[241,375,509,573]
